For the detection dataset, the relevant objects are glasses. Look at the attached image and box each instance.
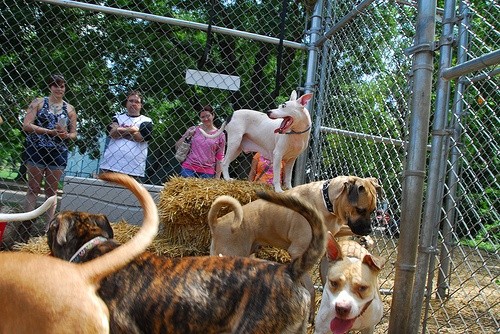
[50,75,64,82]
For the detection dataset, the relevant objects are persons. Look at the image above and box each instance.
[248,152,284,185]
[17,75,76,235]
[99,90,152,183]
[174,105,225,180]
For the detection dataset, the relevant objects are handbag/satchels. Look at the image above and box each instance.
[176,126,196,162]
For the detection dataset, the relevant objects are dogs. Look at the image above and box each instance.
[208,176,381,268]
[315,231,383,334]
[0,195,56,223]
[198,90,313,193]
[0,171,160,334]
[46,190,326,334]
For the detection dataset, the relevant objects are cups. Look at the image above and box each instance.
[58,117,67,130]
